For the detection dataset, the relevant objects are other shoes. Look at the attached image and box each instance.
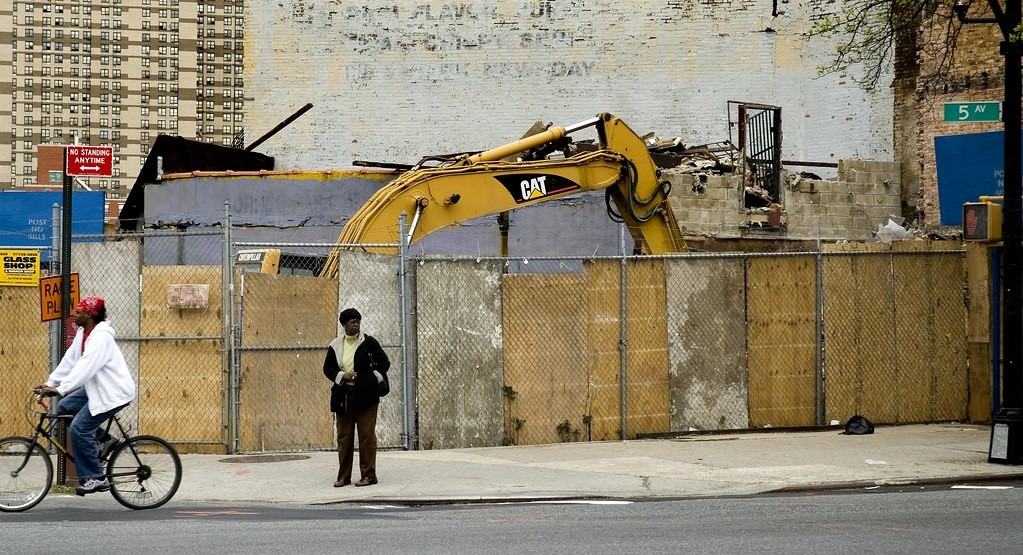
[334,479,351,487]
[355,477,378,486]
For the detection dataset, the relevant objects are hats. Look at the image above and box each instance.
[339,308,361,326]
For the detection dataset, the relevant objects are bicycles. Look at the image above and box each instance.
[0,387,182,513]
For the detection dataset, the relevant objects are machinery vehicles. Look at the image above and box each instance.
[233,112,707,276]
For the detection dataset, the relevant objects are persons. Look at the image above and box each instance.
[323,309,390,487]
[38,297,135,494]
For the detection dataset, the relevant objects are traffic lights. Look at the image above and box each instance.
[961,195,1002,247]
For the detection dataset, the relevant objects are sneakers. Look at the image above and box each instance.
[97,437,119,463]
[75,477,111,494]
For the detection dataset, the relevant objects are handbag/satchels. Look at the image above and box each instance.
[366,335,390,397]
